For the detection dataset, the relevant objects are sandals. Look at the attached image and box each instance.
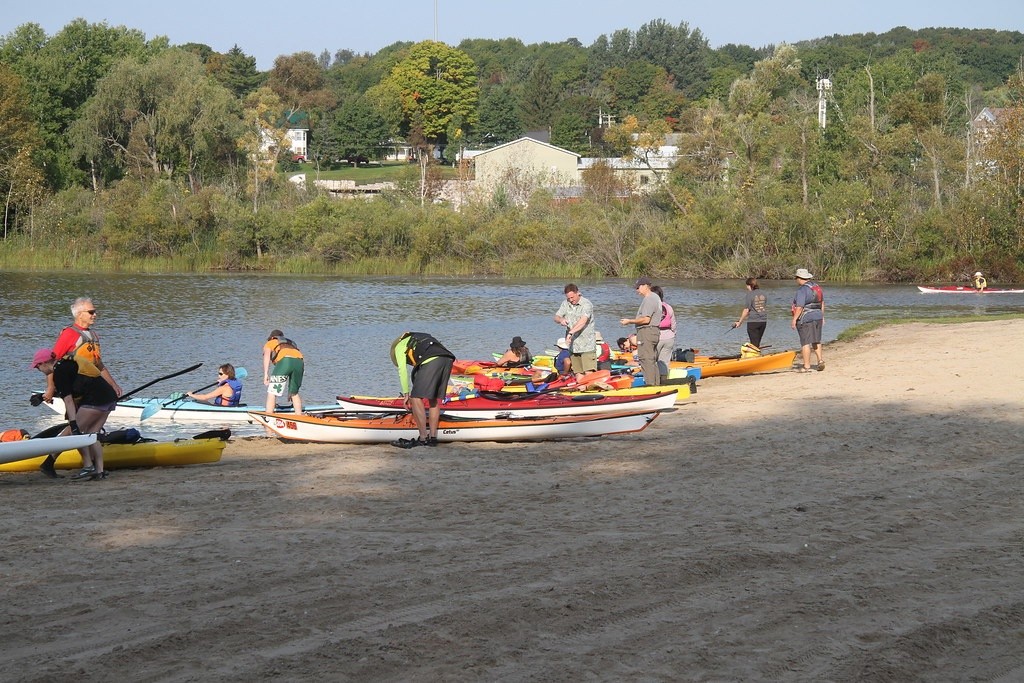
[796,363,812,372]
[817,359,826,371]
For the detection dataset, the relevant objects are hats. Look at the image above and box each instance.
[30,348,55,368]
[390,336,402,367]
[792,269,813,279]
[554,338,568,349]
[510,337,527,348]
[268,330,285,341]
[596,331,603,341]
[636,277,651,286]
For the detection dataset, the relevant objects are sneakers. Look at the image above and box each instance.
[87,473,107,482]
[70,465,96,479]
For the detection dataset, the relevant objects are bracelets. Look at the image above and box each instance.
[568,333,574,336]
[738,320,742,324]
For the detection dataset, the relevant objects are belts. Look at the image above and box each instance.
[661,327,671,330]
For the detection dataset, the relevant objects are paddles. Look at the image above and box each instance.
[33,361,203,439]
[518,370,611,399]
[139,366,248,421]
[709,344,773,359]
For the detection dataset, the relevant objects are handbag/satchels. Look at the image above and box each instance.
[740,341,761,359]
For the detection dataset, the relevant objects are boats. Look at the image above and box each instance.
[0,428,232,471]
[446,349,797,401]
[244,408,659,443]
[0,433,98,464]
[917,285,1024,294]
[30,390,343,420]
[337,389,679,418]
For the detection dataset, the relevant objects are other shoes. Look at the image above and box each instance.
[390,434,439,448]
[38,462,67,478]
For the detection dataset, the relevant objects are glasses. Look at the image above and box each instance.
[79,310,96,316]
[218,372,226,376]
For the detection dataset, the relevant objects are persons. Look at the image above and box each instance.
[263,330,304,415]
[620,277,662,385]
[554,332,637,379]
[38,296,122,479]
[791,268,825,373]
[496,337,532,368]
[735,278,766,349]
[649,286,674,384]
[555,284,596,382]
[30,349,117,481]
[390,332,456,446]
[974,272,986,293]
[188,364,242,407]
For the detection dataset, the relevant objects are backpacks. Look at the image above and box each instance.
[108,428,141,444]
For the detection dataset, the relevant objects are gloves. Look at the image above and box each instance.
[29,393,54,407]
[69,420,83,435]
